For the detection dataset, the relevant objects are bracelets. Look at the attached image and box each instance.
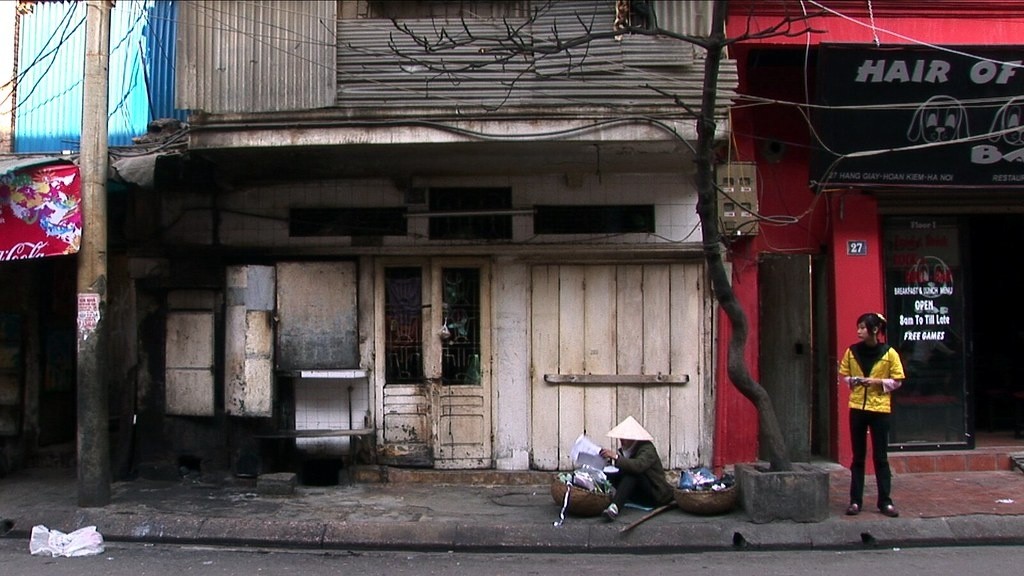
[615,455,618,459]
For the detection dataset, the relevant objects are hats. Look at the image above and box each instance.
[604,415,655,440]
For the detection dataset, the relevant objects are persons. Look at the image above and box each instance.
[838,313,903,517]
[600,417,672,520]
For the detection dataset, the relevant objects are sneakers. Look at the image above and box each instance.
[846,504,859,516]
[880,504,900,518]
[602,503,619,521]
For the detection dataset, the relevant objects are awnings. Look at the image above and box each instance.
[0,152,79,175]
